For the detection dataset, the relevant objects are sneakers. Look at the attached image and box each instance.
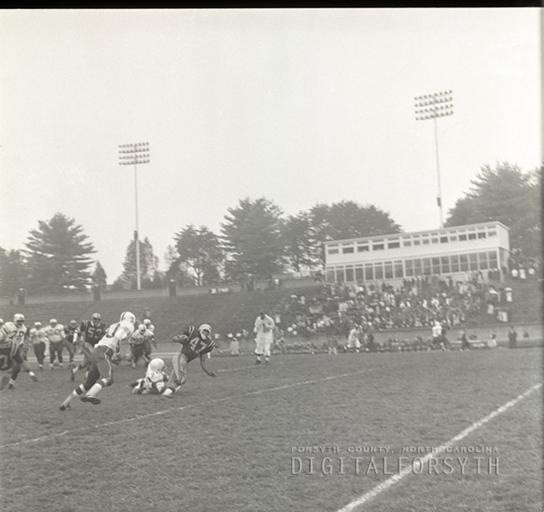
[79,395,100,404]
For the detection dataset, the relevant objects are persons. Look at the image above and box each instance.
[0,267,542,411]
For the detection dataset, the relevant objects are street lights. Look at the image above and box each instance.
[413,89,455,231]
[116,140,151,294]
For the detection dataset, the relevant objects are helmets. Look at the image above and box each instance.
[13,314,25,325]
[34,319,77,329]
[138,319,151,335]
[91,312,102,325]
[120,311,135,324]
[199,324,212,340]
[150,358,165,372]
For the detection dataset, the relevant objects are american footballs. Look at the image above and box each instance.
[180,334,189,341]
